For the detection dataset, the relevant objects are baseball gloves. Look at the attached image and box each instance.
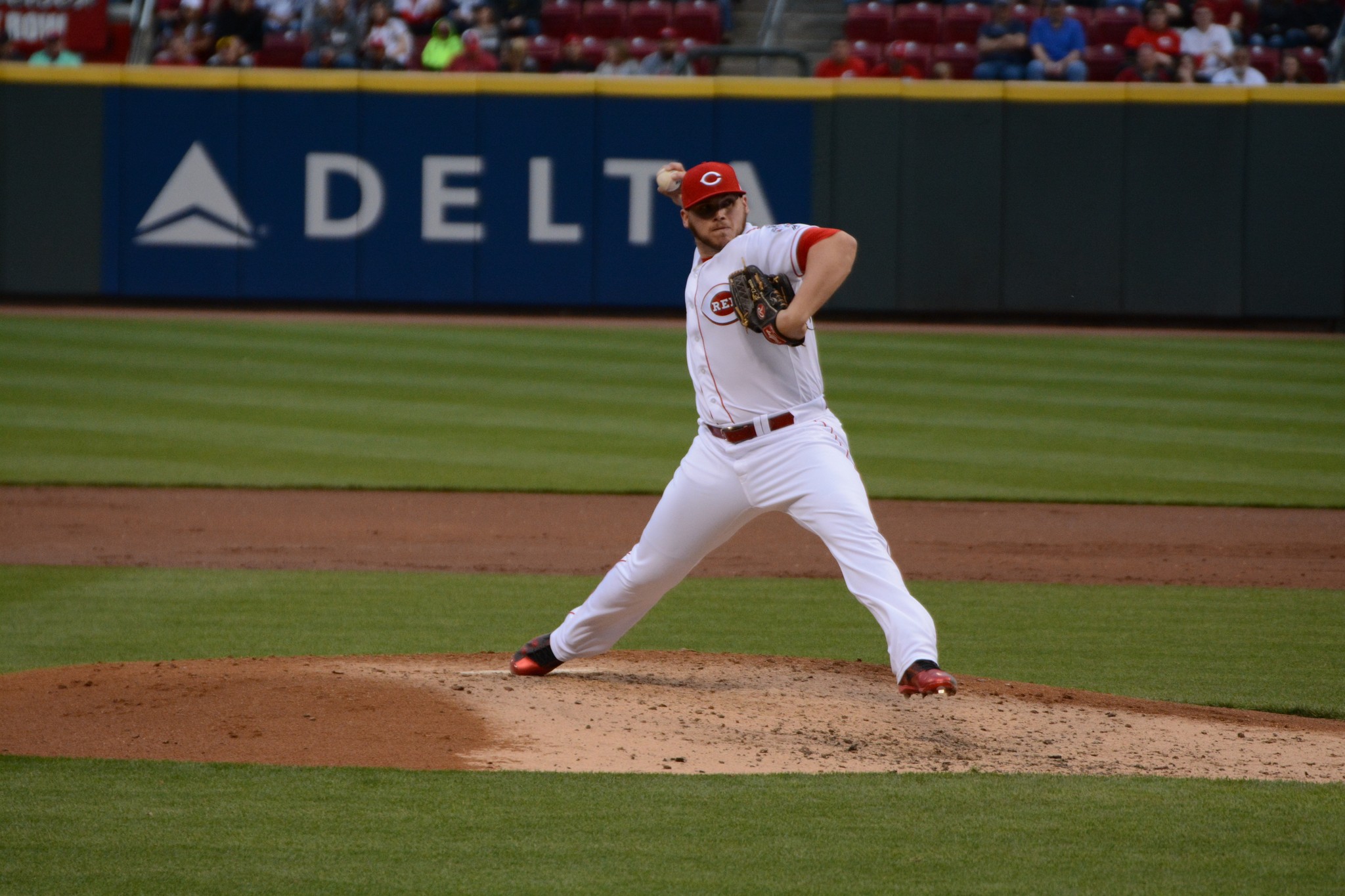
[727,264,806,348]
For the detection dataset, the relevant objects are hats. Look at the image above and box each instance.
[681,161,746,208]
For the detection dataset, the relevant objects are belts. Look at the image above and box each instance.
[707,412,794,444]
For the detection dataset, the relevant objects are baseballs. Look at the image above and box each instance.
[656,166,680,192]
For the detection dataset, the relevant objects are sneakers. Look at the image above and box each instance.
[898,659,958,696]
[511,634,563,677]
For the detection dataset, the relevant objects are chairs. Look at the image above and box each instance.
[256,33,307,71]
[529,0,722,74]
[848,1,1321,86]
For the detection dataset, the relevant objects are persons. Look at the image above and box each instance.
[509,162,962,694]
[0,0,1344,83]
